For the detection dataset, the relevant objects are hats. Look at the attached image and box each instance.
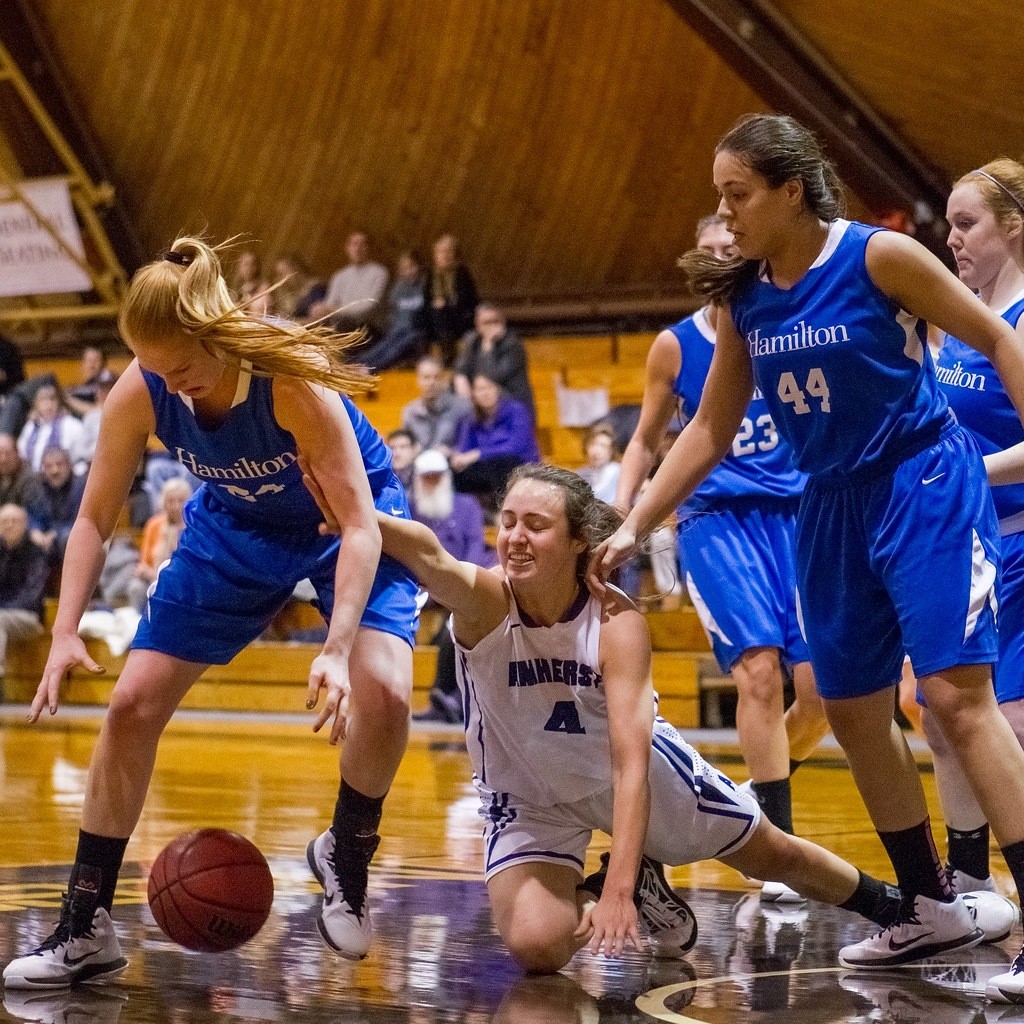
[414,450,449,474]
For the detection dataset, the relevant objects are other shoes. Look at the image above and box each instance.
[429,687,460,722]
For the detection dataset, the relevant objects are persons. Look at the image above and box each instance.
[582,110,1024,1005]
[613,211,833,903]
[1,230,420,991]
[293,435,1021,977]
[1,227,679,724]
[914,154,1024,902]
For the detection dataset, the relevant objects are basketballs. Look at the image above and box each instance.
[146,823,275,954]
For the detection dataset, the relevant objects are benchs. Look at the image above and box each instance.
[0,295,716,749]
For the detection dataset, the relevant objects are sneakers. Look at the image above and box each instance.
[988,942,1024,1003]
[2,990,129,1024]
[737,870,762,888]
[306,826,381,961]
[3,898,127,986]
[760,882,805,902]
[599,852,699,957]
[941,863,1020,937]
[838,893,984,967]
